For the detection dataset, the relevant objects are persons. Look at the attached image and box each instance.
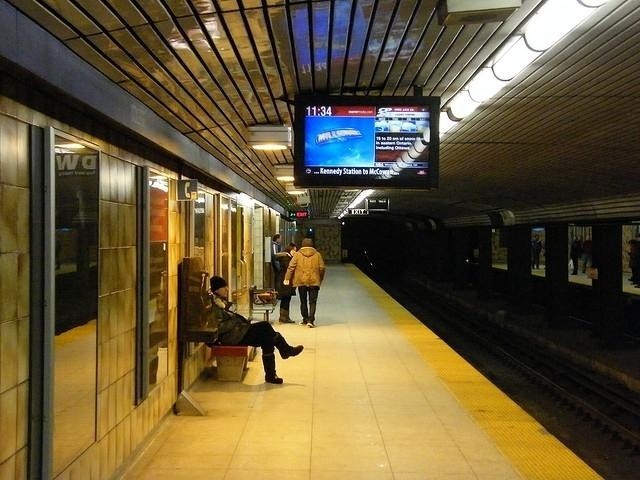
[569,234,581,275]
[628,238,637,283]
[271,233,282,275]
[271,240,299,324]
[282,238,326,328]
[531,233,542,270]
[204,275,304,384]
[631,231,640,289]
[582,234,593,274]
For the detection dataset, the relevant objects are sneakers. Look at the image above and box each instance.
[299,320,315,328]
[278,318,296,323]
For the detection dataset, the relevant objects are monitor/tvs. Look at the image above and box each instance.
[294,93,441,192]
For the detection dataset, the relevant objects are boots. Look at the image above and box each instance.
[272,332,304,359]
[261,354,283,384]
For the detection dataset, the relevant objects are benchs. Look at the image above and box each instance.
[210,320,260,383]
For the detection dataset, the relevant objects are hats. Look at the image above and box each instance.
[210,276,227,293]
